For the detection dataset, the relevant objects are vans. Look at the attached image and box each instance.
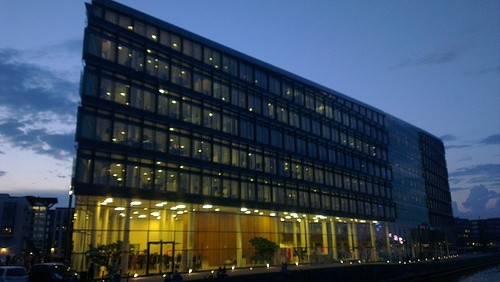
[31,262,80,282]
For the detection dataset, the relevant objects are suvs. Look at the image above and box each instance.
[0,266,29,282]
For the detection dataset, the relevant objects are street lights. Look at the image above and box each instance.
[63,186,74,264]
